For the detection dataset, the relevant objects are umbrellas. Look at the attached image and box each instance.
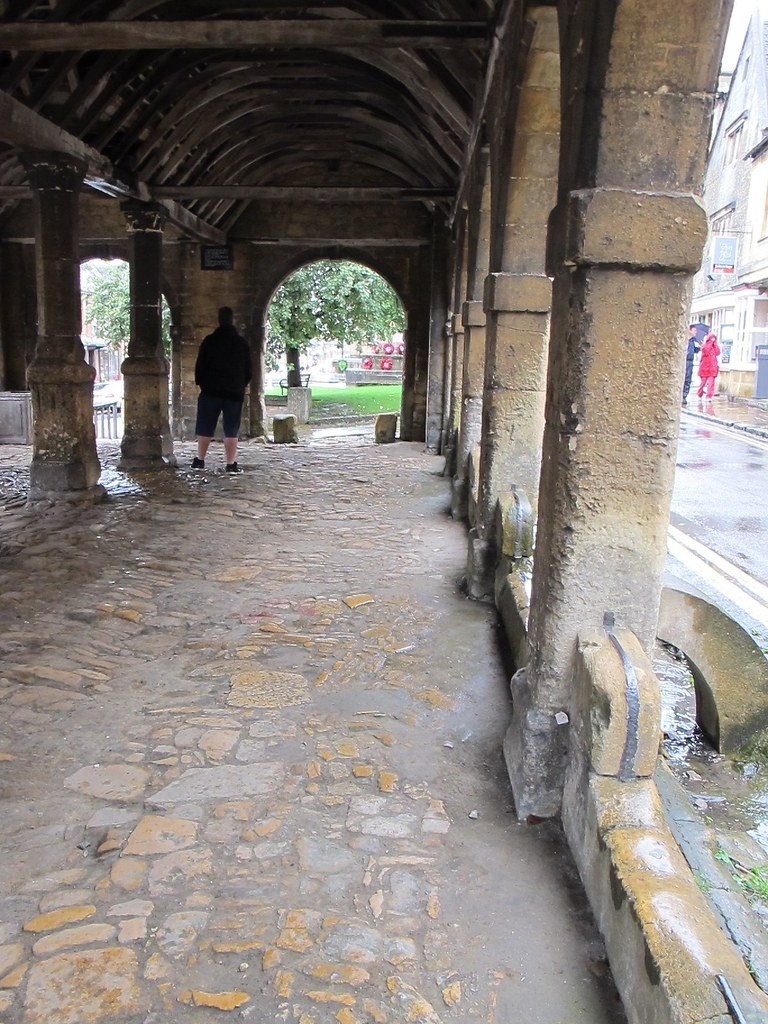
[694,322,709,343]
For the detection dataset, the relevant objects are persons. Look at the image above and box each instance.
[697,333,720,402]
[190,306,252,475]
[682,325,701,404]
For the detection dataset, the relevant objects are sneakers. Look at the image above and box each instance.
[226,462,244,475]
[190,457,204,471]
